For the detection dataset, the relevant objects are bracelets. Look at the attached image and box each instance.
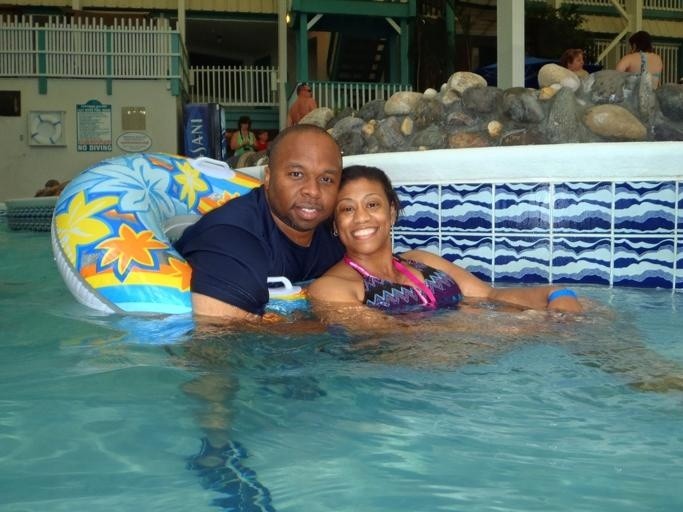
[548,288,577,304]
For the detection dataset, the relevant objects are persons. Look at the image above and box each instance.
[225,116,272,157]
[286,83,318,127]
[307,165,584,314]
[560,49,590,78]
[171,124,347,322]
[615,31,663,88]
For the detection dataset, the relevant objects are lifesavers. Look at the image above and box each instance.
[30,114,62,145]
[51,153,315,314]
